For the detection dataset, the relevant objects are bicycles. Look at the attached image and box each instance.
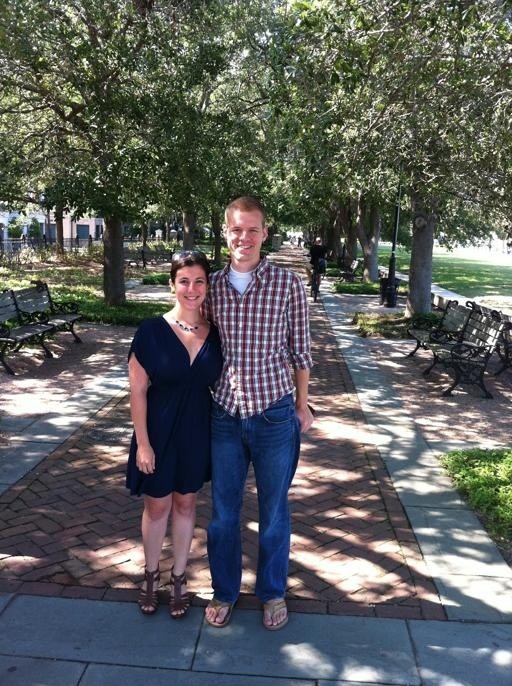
[303,252,322,302]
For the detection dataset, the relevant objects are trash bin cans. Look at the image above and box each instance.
[380,278,401,308]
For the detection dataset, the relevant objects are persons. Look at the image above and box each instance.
[198,197,314,631]
[288,234,316,249]
[127,248,224,620]
[305,237,325,293]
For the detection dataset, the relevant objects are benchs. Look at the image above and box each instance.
[408,301,512,399]
[1,282,83,377]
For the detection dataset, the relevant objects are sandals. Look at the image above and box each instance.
[169,565,190,619]
[205,599,237,627]
[262,598,288,630]
[138,563,160,615]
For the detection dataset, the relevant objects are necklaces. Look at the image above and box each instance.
[172,310,204,331]
